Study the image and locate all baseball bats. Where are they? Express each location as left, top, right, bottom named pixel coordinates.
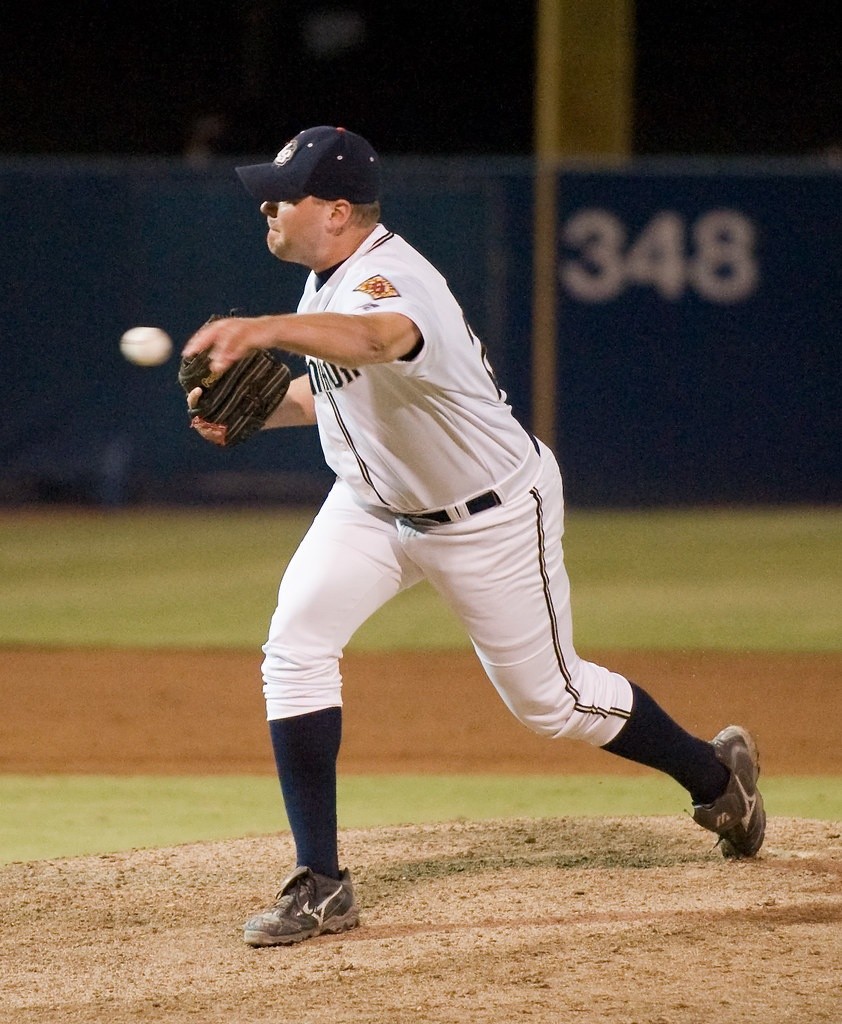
left=176, top=305, right=293, bottom=448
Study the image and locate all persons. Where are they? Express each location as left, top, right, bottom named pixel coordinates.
left=180, top=126, right=767, bottom=948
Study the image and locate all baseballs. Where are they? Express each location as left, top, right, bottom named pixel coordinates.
left=122, top=325, right=172, bottom=363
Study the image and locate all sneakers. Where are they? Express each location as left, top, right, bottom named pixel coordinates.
left=243, top=866, right=361, bottom=946
left=691, top=724, right=766, bottom=859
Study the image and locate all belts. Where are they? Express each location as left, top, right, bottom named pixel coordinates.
left=394, top=433, right=541, bottom=527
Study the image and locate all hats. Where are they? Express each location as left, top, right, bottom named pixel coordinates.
left=237, top=125, right=381, bottom=204
